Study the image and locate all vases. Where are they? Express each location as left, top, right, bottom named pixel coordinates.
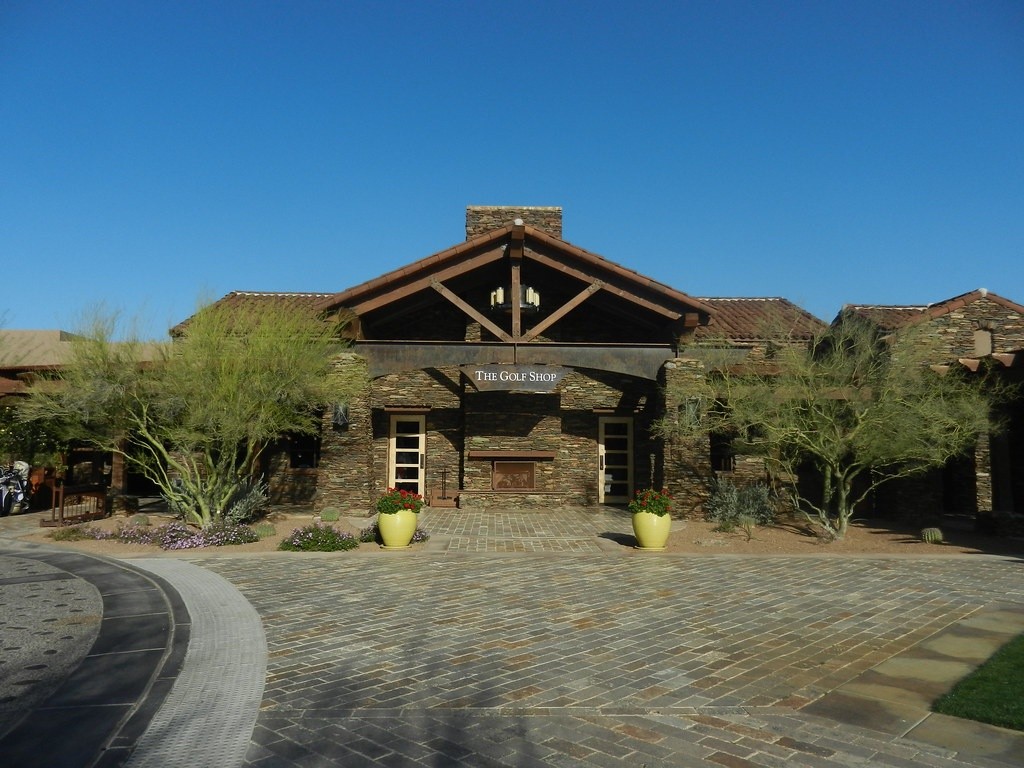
left=631, top=508, right=672, bottom=552
left=378, top=509, right=417, bottom=548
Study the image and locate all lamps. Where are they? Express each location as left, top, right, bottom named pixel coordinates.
left=490, top=286, right=541, bottom=315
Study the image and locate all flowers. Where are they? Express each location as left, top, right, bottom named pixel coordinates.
left=377, top=486, right=424, bottom=515
left=629, top=484, right=675, bottom=516
